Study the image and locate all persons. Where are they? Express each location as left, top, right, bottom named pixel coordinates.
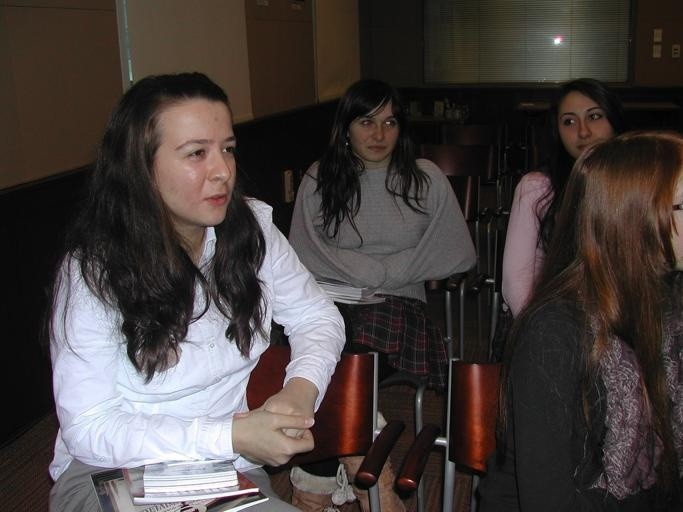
left=470, top=126, right=683, bottom=512
left=287, top=75, right=480, bottom=512
left=500, top=77, right=627, bottom=330
left=47, top=69, right=348, bottom=512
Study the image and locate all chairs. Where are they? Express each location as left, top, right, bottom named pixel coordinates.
left=229, top=98, right=683, bottom=512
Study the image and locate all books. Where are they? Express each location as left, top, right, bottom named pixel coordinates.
left=85, top=455, right=269, bottom=512
left=313, top=276, right=388, bottom=309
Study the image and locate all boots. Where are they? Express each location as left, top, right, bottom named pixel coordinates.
left=358, top=417, right=407, bottom=511
left=289, top=462, right=357, bottom=512
